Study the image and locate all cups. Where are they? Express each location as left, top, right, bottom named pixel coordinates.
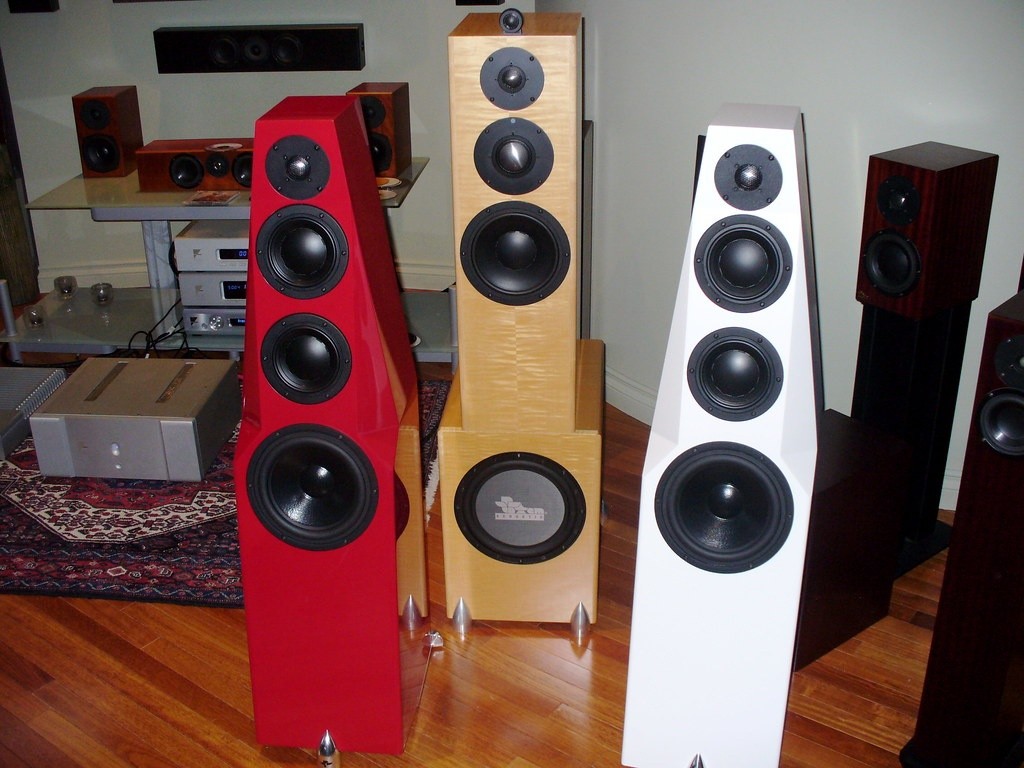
left=90, top=282, right=114, bottom=308
left=23, top=304, right=48, bottom=331
left=53, top=276, right=78, bottom=300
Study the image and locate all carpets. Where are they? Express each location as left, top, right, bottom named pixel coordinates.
left=0, top=379, right=451, bottom=610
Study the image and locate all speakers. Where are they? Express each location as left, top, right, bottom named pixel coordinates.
left=133, top=139, right=250, bottom=195
left=154, top=23, right=366, bottom=74
left=346, top=83, right=412, bottom=174
left=856, top=141, right=999, bottom=322
left=623, top=104, right=825, bottom=768
left=233, top=94, right=425, bottom=751
left=72, top=85, right=142, bottom=179
left=447, top=11, right=585, bottom=432
left=437, top=337, right=604, bottom=629
left=915, top=294, right=1024, bottom=768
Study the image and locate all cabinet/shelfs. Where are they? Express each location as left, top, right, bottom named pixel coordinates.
left=0, top=157, right=459, bottom=375
